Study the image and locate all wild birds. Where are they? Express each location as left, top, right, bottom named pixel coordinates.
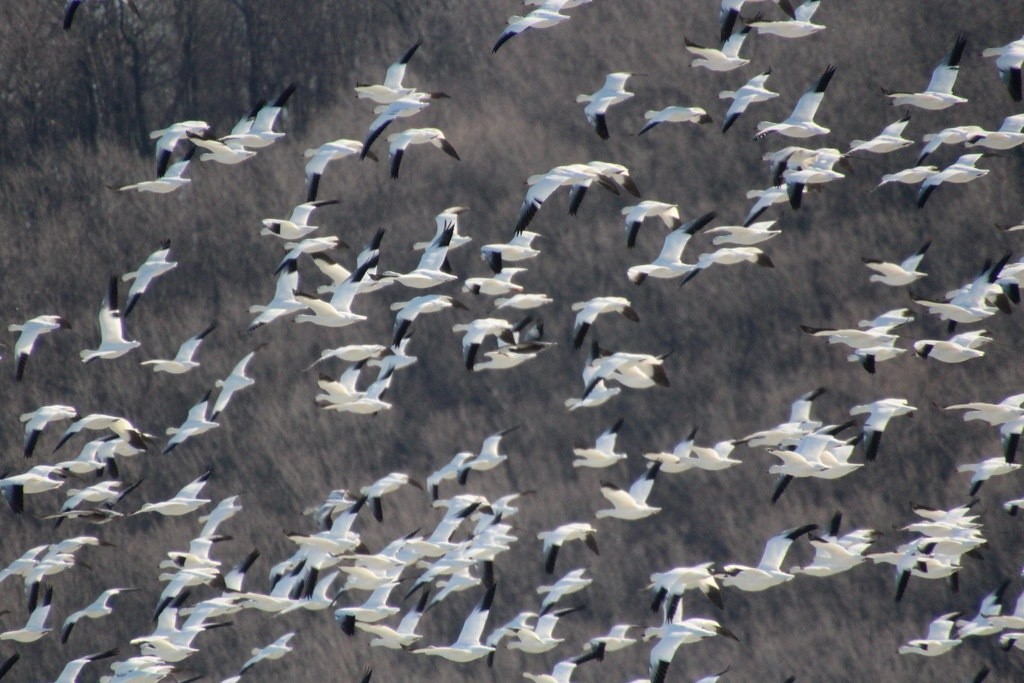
left=0, top=0, right=1023, bottom=683
